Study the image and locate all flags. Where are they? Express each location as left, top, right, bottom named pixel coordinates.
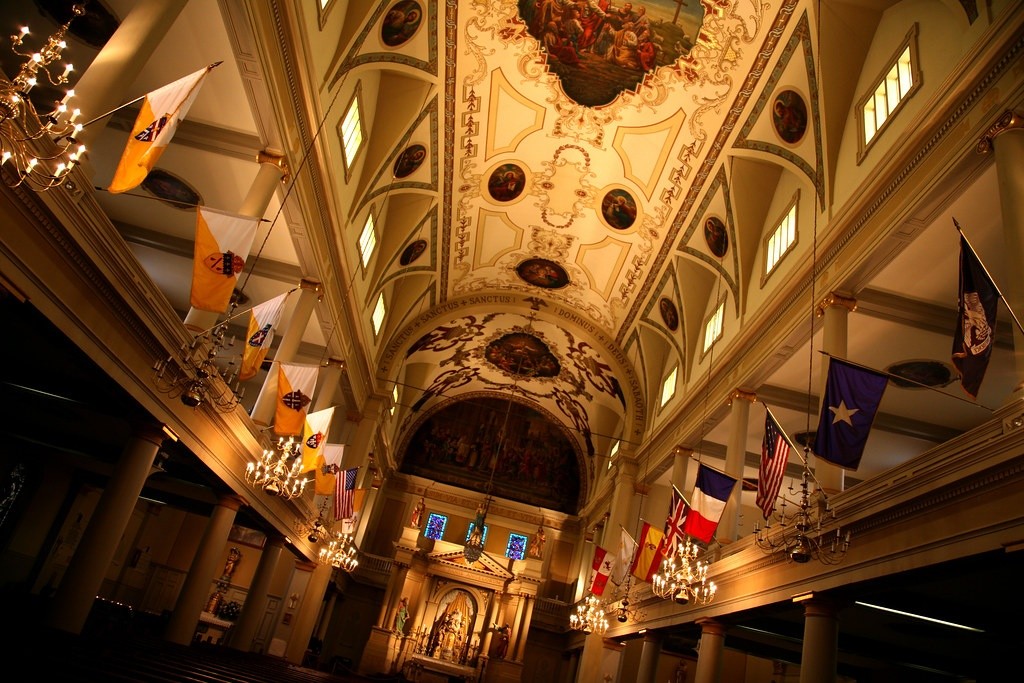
left=240, top=291, right=289, bottom=381
left=312, top=443, right=344, bottom=495
left=659, top=485, right=691, bottom=562
left=684, top=461, right=738, bottom=544
left=609, top=527, right=636, bottom=585
left=106, top=66, right=211, bottom=196
left=189, top=206, right=260, bottom=314
left=587, top=544, right=619, bottom=597
left=301, top=407, right=335, bottom=474
left=330, top=467, right=359, bottom=521
left=274, top=361, right=322, bottom=437
left=630, top=522, right=669, bottom=583
left=950, top=234, right=1000, bottom=401
left=810, top=355, right=890, bottom=472
left=753, top=409, right=792, bottom=522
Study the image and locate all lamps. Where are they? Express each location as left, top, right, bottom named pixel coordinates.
left=566, top=593, right=610, bottom=639
left=149, top=302, right=246, bottom=413
left=0, top=0, right=90, bottom=192
left=749, top=445, right=852, bottom=568
left=320, top=533, right=358, bottom=572
left=245, top=435, right=308, bottom=500
left=653, top=536, right=717, bottom=606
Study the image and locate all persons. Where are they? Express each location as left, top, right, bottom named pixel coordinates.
left=436, top=609, right=466, bottom=651
left=394, top=597, right=410, bottom=635
left=526, top=527, right=546, bottom=558
left=409, top=498, right=425, bottom=529
left=491, top=622, right=512, bottom=661
left=471, top=502, right=488, bottom=541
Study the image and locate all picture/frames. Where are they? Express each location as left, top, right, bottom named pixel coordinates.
left=229, top=525, right=266, bottom=550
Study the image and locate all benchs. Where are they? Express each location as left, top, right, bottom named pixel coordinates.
left=89, top=646, right=372, bottom=683
